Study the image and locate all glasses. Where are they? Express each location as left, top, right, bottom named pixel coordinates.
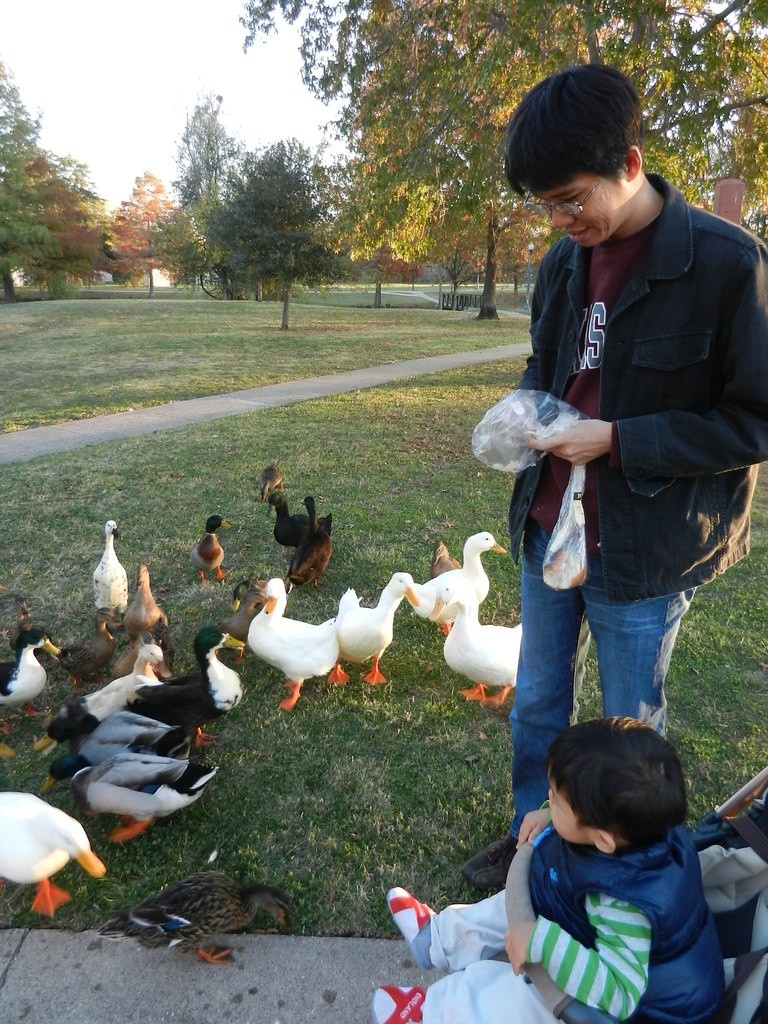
left=522, top=175, right=598, bottom=215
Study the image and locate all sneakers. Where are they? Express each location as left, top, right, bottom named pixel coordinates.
left=461, top=834, right=519, bottom=889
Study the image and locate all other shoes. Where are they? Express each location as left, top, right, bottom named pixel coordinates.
left=386, top=886, right=434, bottom=971
left=371, top=983, right=424, bottom=1024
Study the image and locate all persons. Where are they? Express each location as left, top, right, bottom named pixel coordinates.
left=459, top=63, right=768, bottom=888
left=373, top=715, right=728, bottom=1023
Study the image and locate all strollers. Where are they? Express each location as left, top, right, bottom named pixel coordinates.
left=459, top=764, right=767, bottom=1023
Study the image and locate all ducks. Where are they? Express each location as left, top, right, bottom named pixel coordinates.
left=213, top=570, right=267, bottom=665
left=247, top=578, right=339, bottom=712
left=286, top=495, right=333, bottom=595
left=53, top=608, right=124, bottom=694
left=0, top=589, right=62, bottom=736
left=429, top=575, right=523, bottom=710
left=32, top=698, right=190, bottom=768
left=1, top=790, right=108, bottom=920
left=39, top=642, right=174, bottom=722
left=190, top=514, right=233, bottom=586
left=120, top=563, right=169, bottom=647
left=325, top=571, right=424, bottom=687
left=266, top=490, right=333, bottom=550
left=38, top=753, right=220, bottom=843
left=258, top=463, right=285, bottom=504
left=94, top=869, right=293, bottom=966
left=405, top=531, right=507, bottom=637
left=120, top=626, right=248, bottom=749
left=92, top=519, right=128, bottom=623
